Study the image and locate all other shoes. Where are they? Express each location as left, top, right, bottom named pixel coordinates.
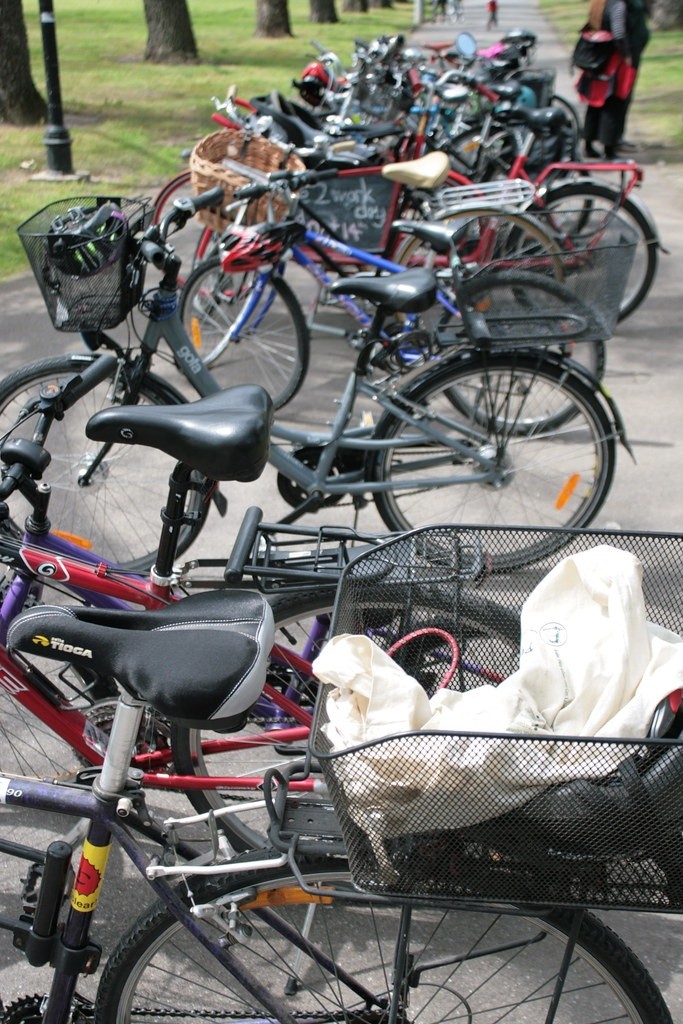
left=604, top=146, right=617, bottom=160
left=585, top=144, right=600, bottom=158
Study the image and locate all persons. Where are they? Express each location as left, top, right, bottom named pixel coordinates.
left=584, top=0, right=649, bottom=163
left=485, top=0, right=498, bottom=32
left=432, top=0, right=463, bottom=23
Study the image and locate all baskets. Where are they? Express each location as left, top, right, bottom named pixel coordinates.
left=16, top=197, right=156, bottom=332
left=306, top=524, right=683, bottom=914
left=188, top=127, right=305, bottom=236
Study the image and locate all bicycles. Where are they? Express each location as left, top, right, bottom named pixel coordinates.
left=0, top=523, right=682, bottom=1024
left=0, top=352, right=525, bottom=869
left=0, top=188, right=635, bottom=574
left=151, top=33, right=670, bottom=437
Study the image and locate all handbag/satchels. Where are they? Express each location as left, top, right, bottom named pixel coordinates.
left=573, top=24, right=618, bottom=76
left=41, top=201, right=129, bottom=326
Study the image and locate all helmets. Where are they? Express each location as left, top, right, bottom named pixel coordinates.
left=521, top=687, right=683, bottom=851
left=298, top=61, right=330, bottom=105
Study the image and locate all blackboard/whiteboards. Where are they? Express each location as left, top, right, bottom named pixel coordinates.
left=299, top=174, right=403, bottom=253
